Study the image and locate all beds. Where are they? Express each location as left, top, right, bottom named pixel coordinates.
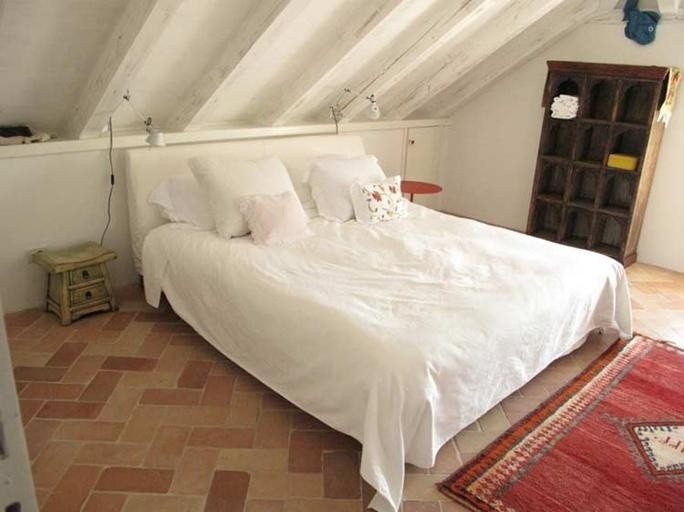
left=119, top=134, right=636, bottom=511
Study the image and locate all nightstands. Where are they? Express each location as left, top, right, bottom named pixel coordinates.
left=31, top=239, right=120, bottom=326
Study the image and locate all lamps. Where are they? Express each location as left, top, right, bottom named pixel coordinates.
left=100, top=91, right=165, bottom=150
left=328, top=87, right=381, bottom=132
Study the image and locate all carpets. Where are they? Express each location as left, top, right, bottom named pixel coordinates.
left=438, top=328, right=684, bottom=511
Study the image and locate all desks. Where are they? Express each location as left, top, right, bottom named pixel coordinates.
left=396, top=179, right=442, bottom=206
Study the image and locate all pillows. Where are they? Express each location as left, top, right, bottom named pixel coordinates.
left=302, top=153, right=387, bottom=223
left=149, top=173, right=214, bottom=231
left=234, top=187, right=315, bottom=246
left=347, top=173, right=410, bottom=225
left=183, top=151, right=310, bottom=241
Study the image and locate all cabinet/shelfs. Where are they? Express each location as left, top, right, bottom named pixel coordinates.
left=524, top=58, right=681, bottom=268
